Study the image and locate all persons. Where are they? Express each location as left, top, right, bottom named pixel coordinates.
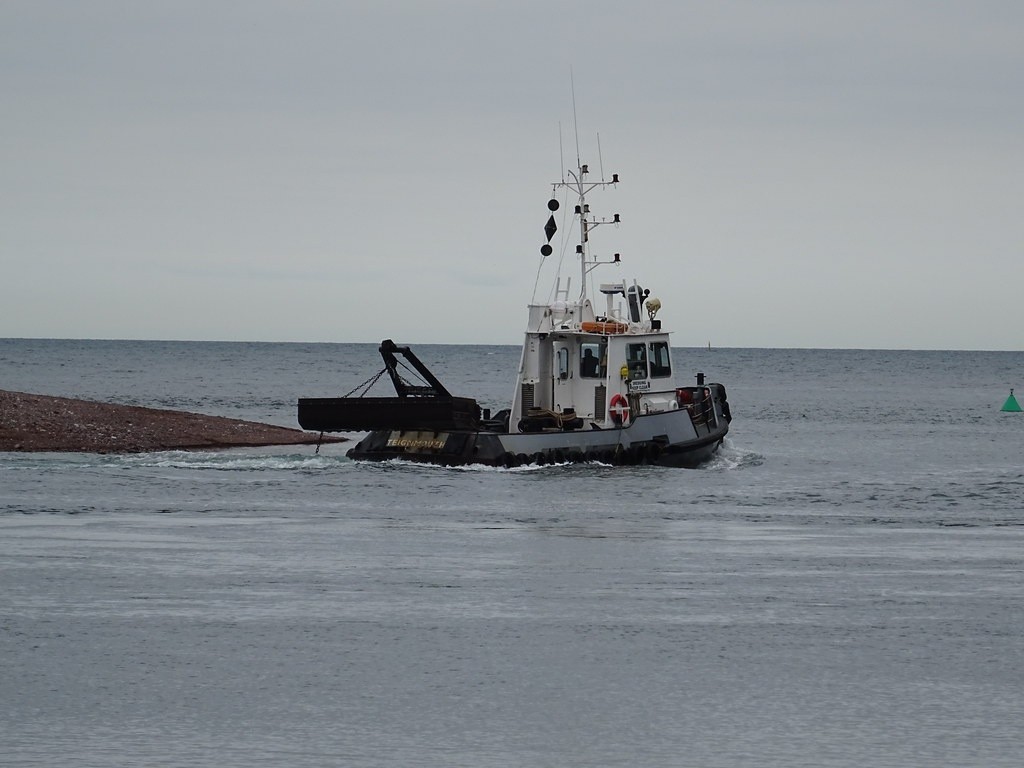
left=582, top=349, right=599, bottom=377
left=640, top=352, right=658, bottom=376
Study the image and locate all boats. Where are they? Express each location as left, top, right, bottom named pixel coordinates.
left=298, top=65, right=732, bottom=467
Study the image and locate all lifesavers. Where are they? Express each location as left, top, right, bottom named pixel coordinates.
left=582, top=320, right=629, bottom=333
left=500, top=441, right=663, bottom=468
left=609, top=394, right=628, bottom=424
